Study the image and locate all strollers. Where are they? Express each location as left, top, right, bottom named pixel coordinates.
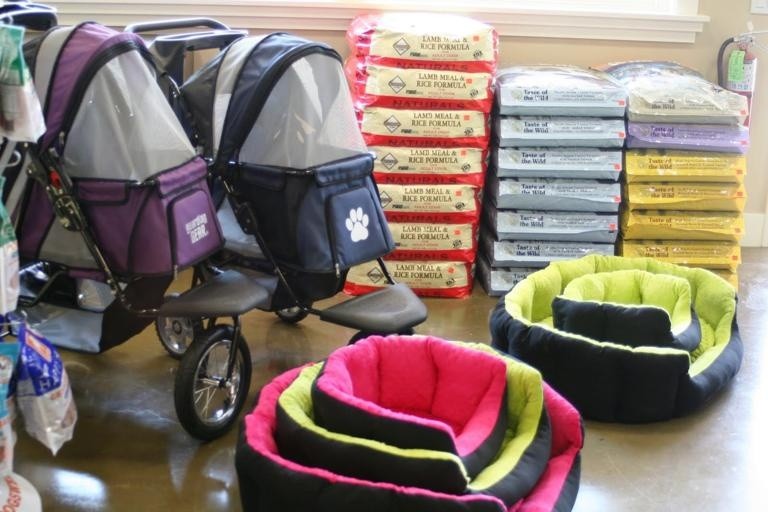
left=124, top=18, right=427, bottom=347
left=0, top=3, right=280, bottom=440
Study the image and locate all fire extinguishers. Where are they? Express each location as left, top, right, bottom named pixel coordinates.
left=717, top=22, right=768, bottom=127
